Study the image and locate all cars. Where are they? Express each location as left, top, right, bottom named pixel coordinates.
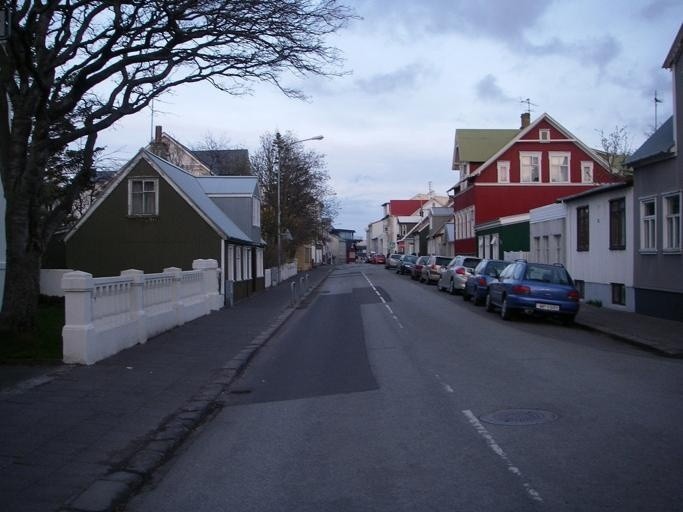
left=384, top=253, right=580, bottom=321
left=368, top=253, right=385, bottom=264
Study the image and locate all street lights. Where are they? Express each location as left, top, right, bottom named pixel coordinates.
left=277, top=135, right=324, bottom=285
